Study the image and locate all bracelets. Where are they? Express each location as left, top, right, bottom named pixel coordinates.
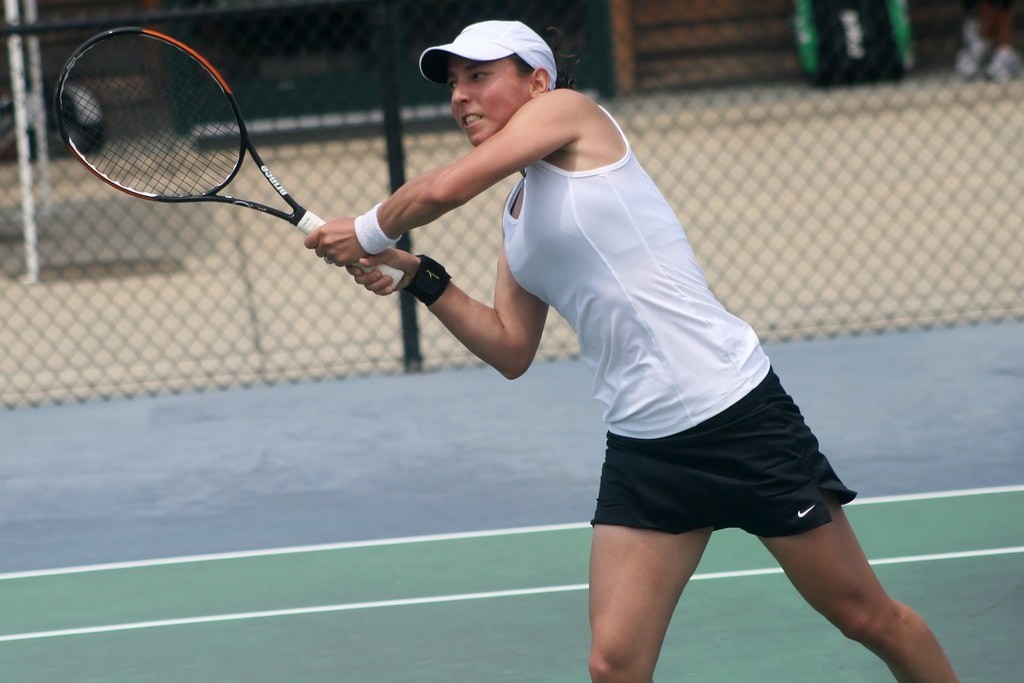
left=354, top=203, right=403, bottom=255
left=403, top=254, right=451, bottom=308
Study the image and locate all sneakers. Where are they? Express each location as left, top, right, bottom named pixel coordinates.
left=954, top=19, right=994, bottom=80
left=985, top=50, right=1021, bottom=82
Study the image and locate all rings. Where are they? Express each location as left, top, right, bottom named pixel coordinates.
left=324, top=256, right=333, bottom=264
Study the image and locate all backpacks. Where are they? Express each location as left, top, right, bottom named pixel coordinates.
left=791, top=0, right=915, bottom=87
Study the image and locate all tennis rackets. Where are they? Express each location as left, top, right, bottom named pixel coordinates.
left=53, top=25, right=406, bottom=293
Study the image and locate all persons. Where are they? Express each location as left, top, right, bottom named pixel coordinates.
left=301, top=20, right=955, bottom=683
left=956, top=0, right=1020, bottom=79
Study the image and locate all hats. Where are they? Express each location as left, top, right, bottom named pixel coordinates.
left=419, top=20, right=557, bottom=91
